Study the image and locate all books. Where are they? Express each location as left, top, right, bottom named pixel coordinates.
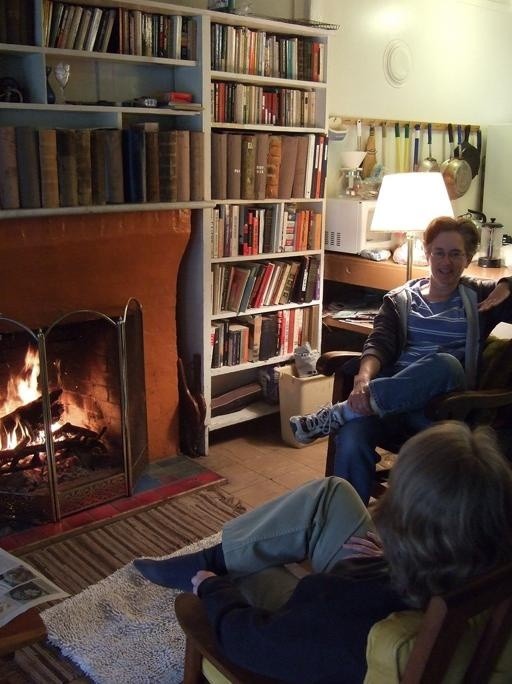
left=0, top=124, right=207, bottom=210
left=210, top=25, right=319, bottom=368
left=0, top=0, right=198, bottom=60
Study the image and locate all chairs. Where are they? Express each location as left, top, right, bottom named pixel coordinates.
left=316, top=322, right=512, bottom=499
left=174, top=565, right=512, bottom=684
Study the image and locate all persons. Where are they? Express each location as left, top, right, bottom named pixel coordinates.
left=133, top=419, right=512, bottom=684
left=290, top=218, right=512, bottom=508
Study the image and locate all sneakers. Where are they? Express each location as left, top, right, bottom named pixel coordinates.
left=289, top=402, right=346, bottom=444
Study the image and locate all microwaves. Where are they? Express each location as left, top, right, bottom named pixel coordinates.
left=325, top=197, right=403, bottom=256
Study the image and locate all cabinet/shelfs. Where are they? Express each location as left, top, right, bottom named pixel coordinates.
left=0, top=1, right=206, bottom=218
left=206, top=13, right=332, bottom=432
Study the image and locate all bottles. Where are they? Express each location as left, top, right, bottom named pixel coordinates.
left=478, top=217, right=504, bottom=268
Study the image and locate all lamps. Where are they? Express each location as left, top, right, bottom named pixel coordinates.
left=370, top=171, right=453, bottom=281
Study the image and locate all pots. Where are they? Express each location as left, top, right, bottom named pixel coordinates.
left=439, top=123, right=456, bottom=174
left=417, top=123, right=440, bottom=171
left=443, top=124, right=473, bottom=201
left=454, top=125, right=480, bottom=179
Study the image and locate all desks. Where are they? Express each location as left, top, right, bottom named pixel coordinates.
left=325, top=255, right=512, bottom=337
left=0, top=607, right=48, bottom=662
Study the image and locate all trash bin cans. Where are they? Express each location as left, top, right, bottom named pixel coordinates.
left=275, top=363, right=336, bottom=448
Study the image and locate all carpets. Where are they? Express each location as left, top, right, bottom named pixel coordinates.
left=10, top=484, right=311, bottom=684
left=40, top=529, right=223, bottom=683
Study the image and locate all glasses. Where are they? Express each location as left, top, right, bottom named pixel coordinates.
left=429, top=250, right=467, bottom=260
